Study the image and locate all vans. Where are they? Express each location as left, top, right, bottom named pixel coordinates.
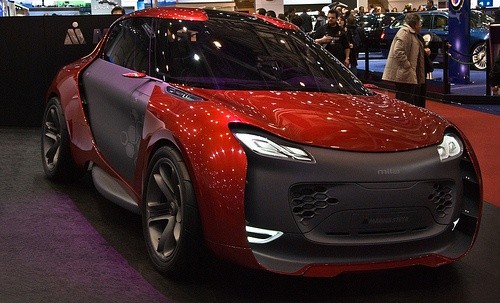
left=361, top=12, right=404, bottom=52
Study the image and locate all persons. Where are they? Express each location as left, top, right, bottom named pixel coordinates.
left=313, top=9, right=351, bottom=67
left=336, top=14, right=358, bottom=78
left=110, top=0, right=500, bottom=31
left=382, top=13, right=431, bottom=110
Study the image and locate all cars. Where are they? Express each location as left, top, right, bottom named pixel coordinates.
left=381, top=10, right=495, bottom=70
left=40, top=6, right=484, bottom=284
